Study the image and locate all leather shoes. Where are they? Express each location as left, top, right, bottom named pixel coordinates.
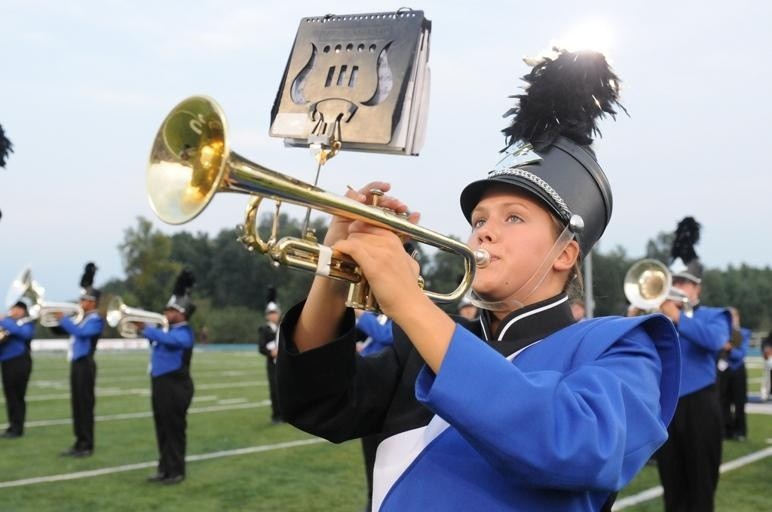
left=162, top=472, right=184, bottom=484
left=60, top=447, right=91, bottom=459
left=147, top=469, right=168, bottom=481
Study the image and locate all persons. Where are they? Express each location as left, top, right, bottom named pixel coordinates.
left=53, top=286, right=104, bottom=457
left=624, top=258, right=732, bottom=512
left=0, top=301, right=33, bottom=438
left=457, top=292, right=480, bottom=320
left=350, top=306, right=395, bottom=512
left=130, top=293, right=194, bottom=483
left=274, top=134, right=679, bottom=512
left=261, top=301, right=287, bottom=421
left=568, top=295, right=585, bottom=320
left=717, top=304, right=754, bottom=438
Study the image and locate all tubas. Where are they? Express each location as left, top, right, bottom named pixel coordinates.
left=0, top=270, right=43, bottom=338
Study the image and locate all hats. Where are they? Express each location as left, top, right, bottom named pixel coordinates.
left=164, top=266, right=196, bottom=318
left=17, top=268, right=38, bottom=310
left=459, top=44, right=618, bottom=258
left=264, top=283, right=281, bottom=315
left=669, top=216, right=704, bottom=284
left=79, top=262, right=100, bottom=301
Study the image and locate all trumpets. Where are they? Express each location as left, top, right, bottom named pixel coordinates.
left=31, top=300, right=84, bottom=330
left=624, top=259, right=694, bottom=322
left=146, top=96, right=490, bottom=317
left=107, top=296, right=170, bottom=338
